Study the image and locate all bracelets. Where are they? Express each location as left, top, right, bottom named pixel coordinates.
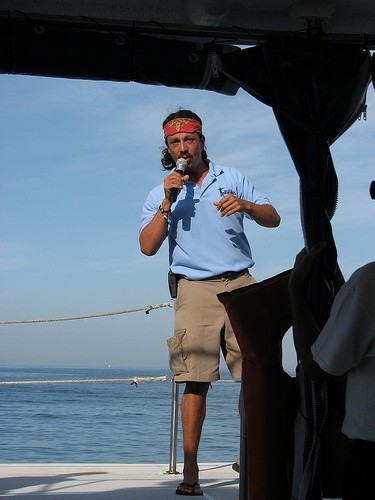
left=159, top=206, right=172, bottom=224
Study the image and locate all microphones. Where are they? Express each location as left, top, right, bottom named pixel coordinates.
left=168, top=157, right=188, bottom=203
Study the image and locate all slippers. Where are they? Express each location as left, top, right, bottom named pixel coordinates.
left=175, top=482, right=203, bottom=496
left=232, top=463, right=239, bottom=473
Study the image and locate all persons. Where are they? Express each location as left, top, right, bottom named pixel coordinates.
left=138, top=110, right=281, bottom=495
left=289, top=240, right=375, bottom=500
